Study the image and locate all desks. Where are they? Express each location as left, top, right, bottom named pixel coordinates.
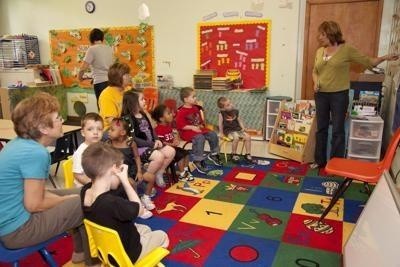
left=0, top=119, right=83, bottom=190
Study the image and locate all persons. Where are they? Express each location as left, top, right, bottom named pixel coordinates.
left=153, top=104, right=195, bottom=182
left=121, top=90, right=176, bottom=188
left=76, top=28, right=114, bottom=112
left=107, top=117, right=157, bottom=220
left=80, top=142, right=169, bottom=267
left=175, top=87, right=224, bottom=175
left=0, top=91, right=101, bottom=267
left=71, top=112, right=105, bottom=189
left=310, top=20, right=399, bottom=169
left=97, top=62, right=132, bottom=137
left=218, top=96, right=253, bottom=164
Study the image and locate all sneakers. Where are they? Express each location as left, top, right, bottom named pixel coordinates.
left=71, top=252, right=85, bottom=263
left=140, top=208, right=153, bottom=220
left=208, top=154, right=223, bottom=166
left=177, top=174, right=188, bottom=181
left=141, top=196, right=155, bottom=211
left=192, top=161, right=206, bottom=174
left=244, top=153, right=252, bottom=163
left=231, top=153, right=239, bottom=162
left=185, top=170, right=194, bottom=181
left=155, top=173, right=166, bottom=188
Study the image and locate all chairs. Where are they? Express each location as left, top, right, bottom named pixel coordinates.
left=83, top=219, right=170, bottom=267
left=0, top=231, right=69, bottom=267
left=318, top=131, right=400, bottom=222
left=62, top=157, right=74, bottom=188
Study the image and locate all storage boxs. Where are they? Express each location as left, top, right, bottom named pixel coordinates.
left=0, top=68, right=56, bottom=86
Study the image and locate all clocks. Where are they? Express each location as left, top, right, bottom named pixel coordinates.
left=85, top=1, right=95, bottom=13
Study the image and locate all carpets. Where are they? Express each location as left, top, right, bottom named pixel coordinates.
left=0, top=149, right=399, bottom=267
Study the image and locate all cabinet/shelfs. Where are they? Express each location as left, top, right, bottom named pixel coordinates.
left=346, top=111, right=384, bottom=162
left=268, top=101, right=320, bottom=165
left=266, top=96, right=292, bottom=141
left=158, top=86, right=269, bottom=137
left=0, top=84, right=67, bottom=126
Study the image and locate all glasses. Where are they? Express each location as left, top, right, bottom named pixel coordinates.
left=53, top=115, right=64, bottom=123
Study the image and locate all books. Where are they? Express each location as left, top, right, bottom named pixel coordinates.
left=211, top=77, right=233, bottom=91
